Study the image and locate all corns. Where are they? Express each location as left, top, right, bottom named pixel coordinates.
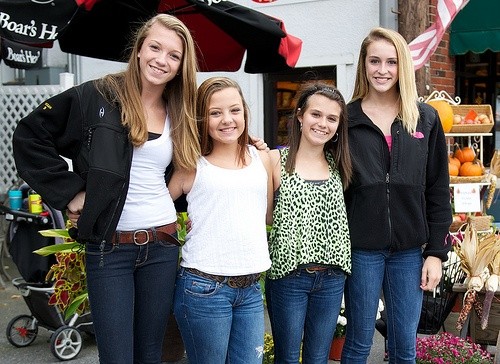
left=486, top=180, right=496, bottom=209
left=454, top=289, right=500, bottom=331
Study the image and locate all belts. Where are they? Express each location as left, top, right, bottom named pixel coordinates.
left=301, top=264, right=329, bottom=274
left=184, top=268, right=261, bottom=290
left=112, top=221, right=183, bottom=252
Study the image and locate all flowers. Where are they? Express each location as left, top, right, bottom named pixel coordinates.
left=333, top=307, right=347, bottom=337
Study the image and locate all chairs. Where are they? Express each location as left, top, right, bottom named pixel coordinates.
left=376, top=258, right=466, bottom=361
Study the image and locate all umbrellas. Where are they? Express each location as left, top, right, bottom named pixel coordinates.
left=0, top=0, right=303, bottom=76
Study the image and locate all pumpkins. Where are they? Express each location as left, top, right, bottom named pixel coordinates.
left=428, top=101, right=454, bottom=133
left=448, top=143, right=483, bottom=176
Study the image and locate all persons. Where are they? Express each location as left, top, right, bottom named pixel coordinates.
left=182, top=83, right=352, bottom=364
left=326, top=27, right=453, bottom=364
left=65, top=77, right=274, bottom=364
left=12, top=13, right=271, bottom=364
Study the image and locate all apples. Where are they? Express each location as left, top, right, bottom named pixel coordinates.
left=454, top=114, right=491, bottom=124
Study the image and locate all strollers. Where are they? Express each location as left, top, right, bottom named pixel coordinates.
left=0, top=184, right=96, bottom=361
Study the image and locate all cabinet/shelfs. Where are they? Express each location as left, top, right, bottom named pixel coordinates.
left=276, top=87, right=294, bottom=144
left=417, top=89, right=495, bottom=241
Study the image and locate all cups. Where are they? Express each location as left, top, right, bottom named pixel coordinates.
left=8, top=190, right=22, bottom=210
left=28, top=194, right=42, bottom=214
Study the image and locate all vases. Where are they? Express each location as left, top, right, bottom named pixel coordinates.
left=329, top=336, right=346, bottom=360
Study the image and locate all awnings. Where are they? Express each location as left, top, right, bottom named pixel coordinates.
left=449, top=0, right=500, bottom=55
